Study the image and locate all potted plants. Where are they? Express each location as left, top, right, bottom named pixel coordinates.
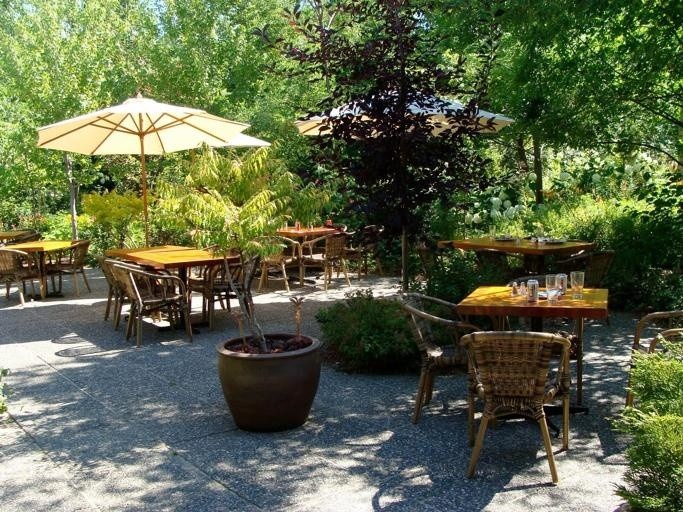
left=148, top=138, right=325, bottom=434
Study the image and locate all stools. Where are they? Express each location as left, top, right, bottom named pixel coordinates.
left=624, top=304, right=683, bottom=420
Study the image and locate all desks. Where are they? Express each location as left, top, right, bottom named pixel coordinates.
left=452, top=285, right=610, bottom=442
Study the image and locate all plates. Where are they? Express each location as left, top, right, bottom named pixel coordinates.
left=494, top=238, right=517, bottom=241
left=542, top=239, right=567, bottom=244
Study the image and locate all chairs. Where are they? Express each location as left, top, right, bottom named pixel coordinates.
left=416, top=236, right=614, bottom=289
left=454, top=329, right=577, bottom=489
left=501, top=272, right=594, bottom=408
left=94, top=244, right=261, bottom=348
left=0, top=230, right=91, bottom=306
left=247, top=223, right=384, bottom=293
left=394, top=291, right=486, bottom=428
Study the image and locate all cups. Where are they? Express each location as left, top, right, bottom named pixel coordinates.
left=280, top=221, right=287, bottom=231
left=570, top=271, right=584, bottom=299
left=489, top=225, right=496, bottom=239
left=325, top=220, right=331, bottom=228
left=306, top=221, right=312, bottom=231
left=545, top=273, right=559, bottom=303
left=294, top=219, right=300, bottom=231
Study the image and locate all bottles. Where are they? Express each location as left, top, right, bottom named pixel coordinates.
left=511, top=282, right=518, bottom=296
left=519, top=282, right=526, bottom=297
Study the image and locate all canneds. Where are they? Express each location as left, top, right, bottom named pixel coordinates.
left=527, top=280, right=539, bottom=302
left=295, top=222, right=301, bottom=231
left=555, top=273, right=567, bottom=293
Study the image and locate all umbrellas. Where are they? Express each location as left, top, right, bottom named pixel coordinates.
left=34, top=92, right=252, bottom=248
left=293, top=90, right=516, bottom=138
left=223, top=133, right=272, bottom=147
